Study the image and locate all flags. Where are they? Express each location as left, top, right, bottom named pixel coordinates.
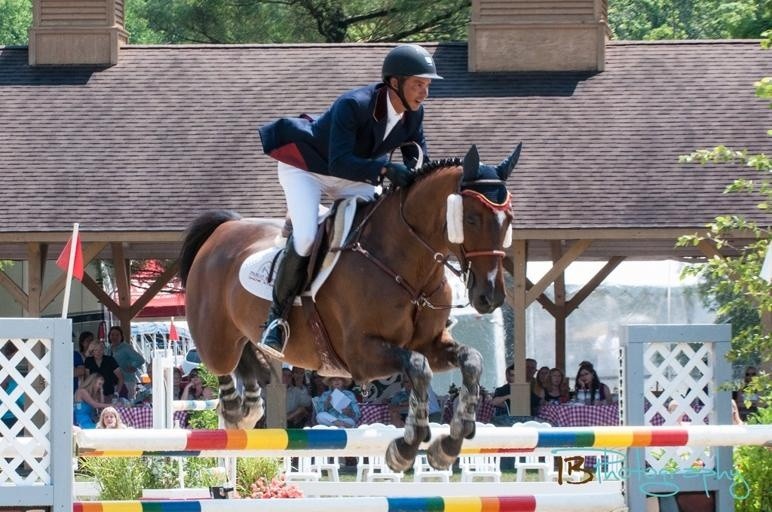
left=55, top=232, right=85, bottom=282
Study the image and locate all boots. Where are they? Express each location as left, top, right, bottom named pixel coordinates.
left=260, top=236, right=310, bottom=351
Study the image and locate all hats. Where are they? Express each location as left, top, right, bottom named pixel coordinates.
left=322, top=375, right=353, bottom=388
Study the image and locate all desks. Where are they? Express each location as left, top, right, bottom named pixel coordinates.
left=114, top=379, right=708, bottom=428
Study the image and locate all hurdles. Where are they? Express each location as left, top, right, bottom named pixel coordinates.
left=0, top=426, right=772, bottom=511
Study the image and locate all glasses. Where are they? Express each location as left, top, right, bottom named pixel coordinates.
left=190, top=373, right=202, bottom=378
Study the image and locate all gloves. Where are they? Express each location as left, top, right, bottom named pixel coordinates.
left=384, top=163, right=415, bottom=186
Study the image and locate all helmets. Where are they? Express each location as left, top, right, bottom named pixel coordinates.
left=382, top=44, right=444, bottom=80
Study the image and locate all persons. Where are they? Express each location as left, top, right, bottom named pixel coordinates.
left=72, top=326, right=765, bottom=432
left=255, top=44, right=445, bottom=357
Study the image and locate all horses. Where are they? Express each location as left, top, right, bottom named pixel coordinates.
left=177, top=140, right=524, bottom=474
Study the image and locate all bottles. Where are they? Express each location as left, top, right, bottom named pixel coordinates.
left=577, top=389, right=600, bottom=406
left=111, top=386, right=119, bottom=405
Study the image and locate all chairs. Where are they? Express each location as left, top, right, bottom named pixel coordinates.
left=284, top=422, right=585, bottom=485
